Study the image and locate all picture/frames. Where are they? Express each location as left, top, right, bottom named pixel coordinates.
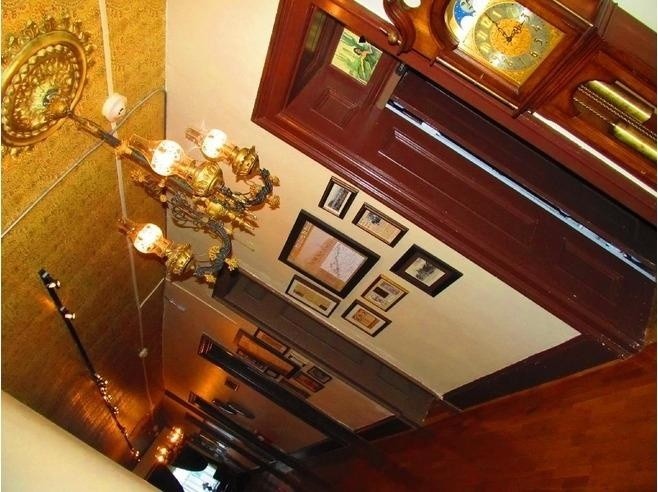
left=232, top=176, right=464, bottom=401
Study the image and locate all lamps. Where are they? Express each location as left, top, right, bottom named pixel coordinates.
left=146, top=425, right=187, bottom=467
left=1, top=15, right=279, bottom=287
left=38, top=267, right=141, bottom=462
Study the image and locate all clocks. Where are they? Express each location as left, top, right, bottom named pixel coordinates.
left=383, top=0, right=657, bottom=225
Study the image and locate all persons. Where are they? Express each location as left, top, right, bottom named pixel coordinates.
left=354, top=46, right=375, bottom=82
